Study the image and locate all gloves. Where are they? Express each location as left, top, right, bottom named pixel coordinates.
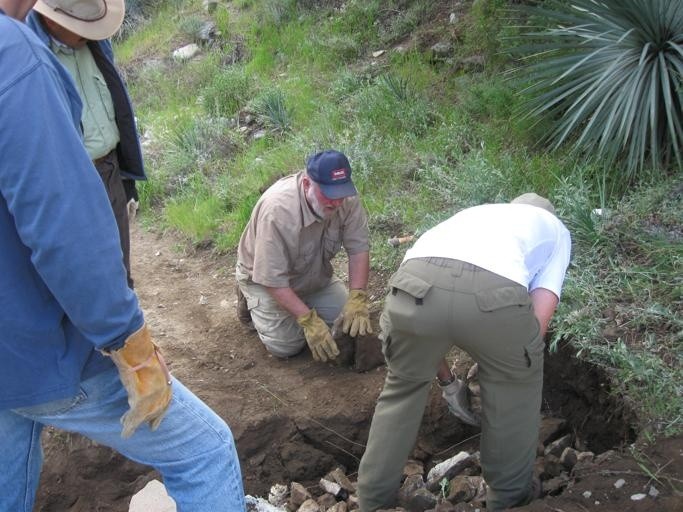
left=294, top=308, right=340, bottom=362
left=95, top=321, right=173, bottom=439
left=438, top=363, right=480, bottom=428
left=333, top=289, right=373, bottom=335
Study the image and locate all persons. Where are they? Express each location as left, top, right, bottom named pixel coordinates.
left=355, top=191, right=573, bottom=511
left=21, top=2, right=149, bottom=293
left=0, top=1, right=248, bottom=512
left=232, top=149, right=378, bottom=365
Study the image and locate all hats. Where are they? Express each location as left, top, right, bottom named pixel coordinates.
left=509, top=191, right=557, bottom=213
left=31, top=1, right=125, bottom=40
left=306, top=149, right=357, bottom=201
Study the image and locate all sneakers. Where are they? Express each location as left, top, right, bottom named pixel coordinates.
left=532, top=474, right=541, bottom=500
left=333, top=329, right=386, bottom=370
left=233, top=283, right=252, bottom=323
